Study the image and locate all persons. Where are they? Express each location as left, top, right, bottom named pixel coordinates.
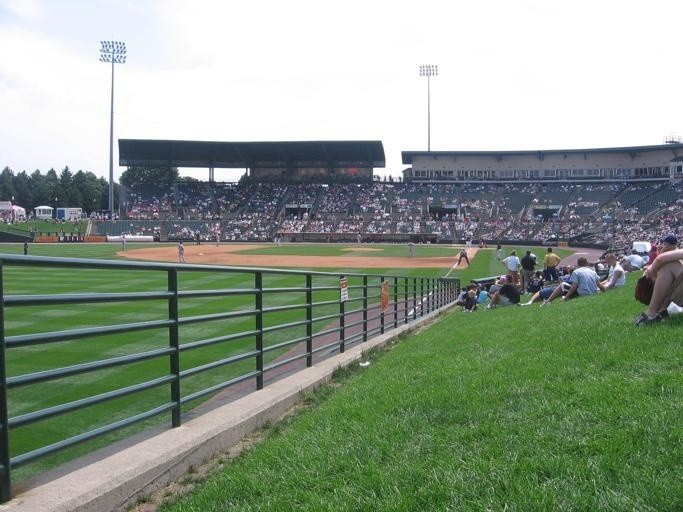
left=178, top=241, right=186, bottom=263
left=634, top=236, right=683, bottom=326
left=0, top=181, right=683, bottom=252
left=24, top=241, right=29, bottom=255
left=407, top=240, right=415, bottom=257
left=457, top=244, right=644, bottom=312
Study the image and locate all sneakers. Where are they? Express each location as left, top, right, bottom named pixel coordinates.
left=635, top=301, right=683, bottom=325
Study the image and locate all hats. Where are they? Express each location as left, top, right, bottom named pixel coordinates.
left=662, top=236, right=676, bottom=244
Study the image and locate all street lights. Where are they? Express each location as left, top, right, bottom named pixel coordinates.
left=418, top=64, right=439, bottom=152
left=93, top=197, right=96, bottom=212
left=11, top=195, right=15, bottom=207
left=95, top=37, right=128, bottom=218
left=54, top=196, right=57, bottom=209
